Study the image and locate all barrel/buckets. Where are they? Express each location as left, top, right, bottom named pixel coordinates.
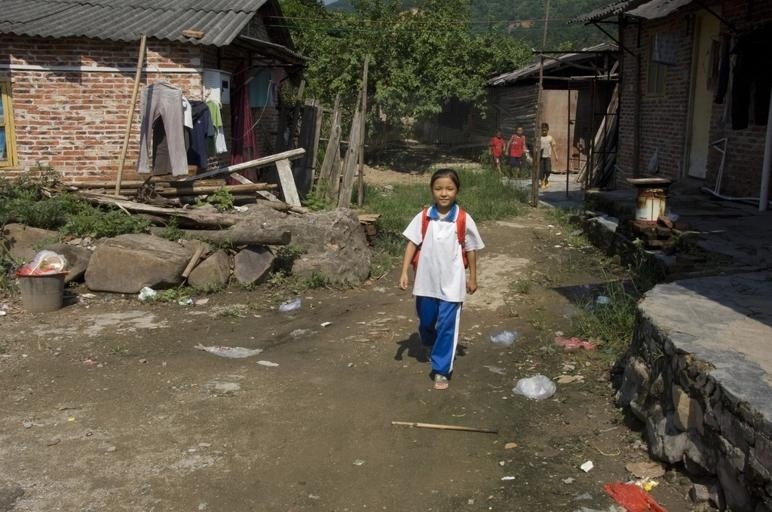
left=16, top=262, right=71, bottom=313
left=635, top=186, right=671, bottom=222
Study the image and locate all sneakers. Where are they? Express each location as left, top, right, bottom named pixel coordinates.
left=431, top=373, right=449, bottom=390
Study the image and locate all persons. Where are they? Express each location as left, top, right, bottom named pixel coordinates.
left=538, top=123, right=558, bottom=189
left=399, top=169, right=485, bottom=390
left=488, top=131, right=506, bottom=177
left=505, top=127, right=528, bottom=179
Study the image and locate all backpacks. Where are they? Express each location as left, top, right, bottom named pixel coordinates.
left=412, top=207, right=468, bottom=271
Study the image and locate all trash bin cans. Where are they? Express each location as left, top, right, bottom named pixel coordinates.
left=17, top=272, right=68, bottom=313
